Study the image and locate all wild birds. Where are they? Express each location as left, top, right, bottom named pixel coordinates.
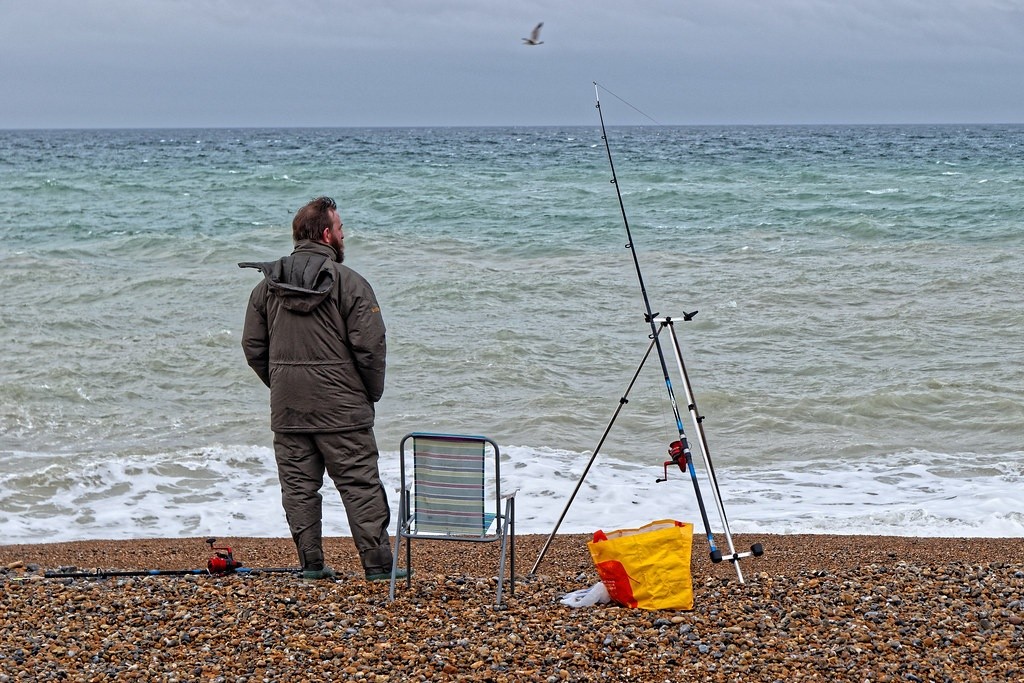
left=521, top=22, right=545, bottom=45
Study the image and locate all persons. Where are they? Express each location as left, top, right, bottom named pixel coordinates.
left=241, top=196, right=415, bottom=582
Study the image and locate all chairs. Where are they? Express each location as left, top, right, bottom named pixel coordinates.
left=389, top=432, right=520, bottom=605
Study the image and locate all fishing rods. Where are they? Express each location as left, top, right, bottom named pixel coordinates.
left=42, top=539, right=308, bottom=579
left=593, top=83, right=721, bottom=562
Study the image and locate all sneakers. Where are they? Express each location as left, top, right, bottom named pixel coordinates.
left=365, top=566, right=415, bottom=581
left=303, top=565, right=336, bottom=580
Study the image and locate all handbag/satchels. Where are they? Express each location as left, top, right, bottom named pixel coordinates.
left=587, top=519, right=694, bottom=611
